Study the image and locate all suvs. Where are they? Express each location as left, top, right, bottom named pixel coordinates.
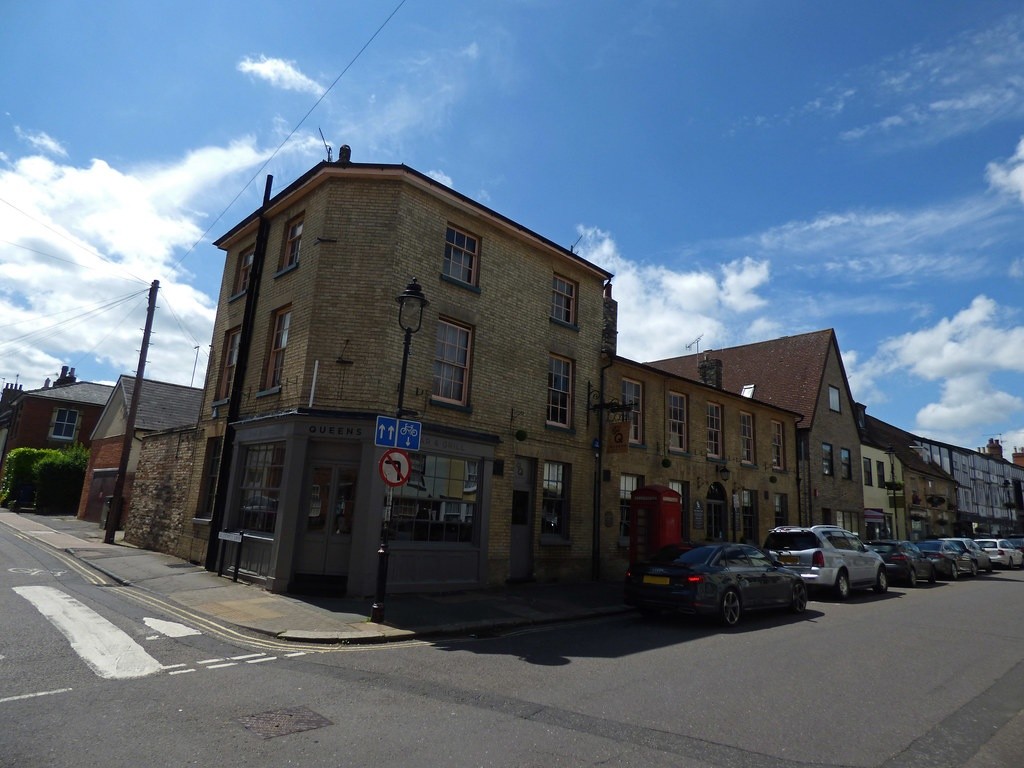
left=761, top=522, right=889, bottom=602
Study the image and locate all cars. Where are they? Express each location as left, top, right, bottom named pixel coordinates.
left=974, top=538, right=1024, bottom=570
left=938, top=537, right=992, bottom=573
left=913, top=540, right=979, bottom=581
left=861, top=539, right=940, bottom=588
left=622, top=539, right=809, bottom=627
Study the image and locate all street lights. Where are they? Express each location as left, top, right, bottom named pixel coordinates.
left=999, top=480, right=1013, bottom=535
left=369, top=277, right=431, bottom=623
left=884, top=444, right=903, bottom=539
